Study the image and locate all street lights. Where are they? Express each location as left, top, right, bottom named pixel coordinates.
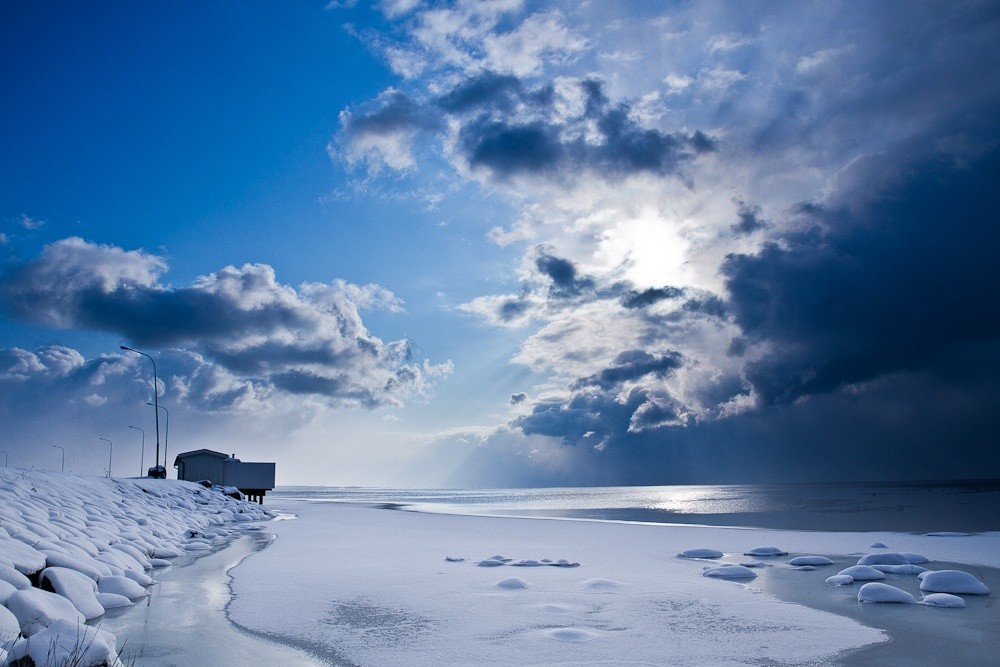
left=99, top=438, right=112, bottom=479
left=146, top=403, right=168, bottom=467
left=120, top=346, right=159, bottom=467
left=129, top=426, right=144, bottom=477
left=53, top=446, right=64, bottom=472
